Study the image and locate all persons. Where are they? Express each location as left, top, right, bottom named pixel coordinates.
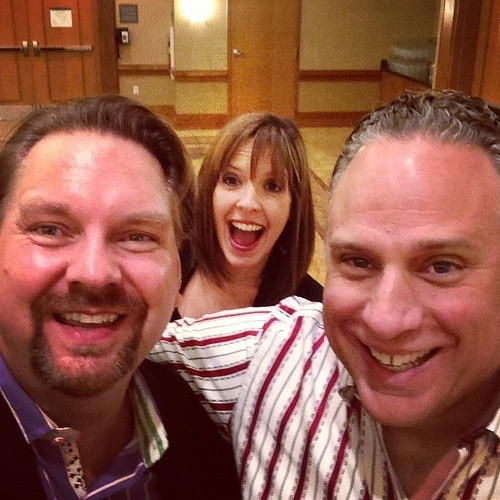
left=167, top=109, right=327, bottom=325
left=142, top=87, right=499, bottom=500
left=0, top=94, right=243, bottom=500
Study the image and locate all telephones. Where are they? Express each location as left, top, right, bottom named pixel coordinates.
left=115, top=26, right=131, bottom=45
